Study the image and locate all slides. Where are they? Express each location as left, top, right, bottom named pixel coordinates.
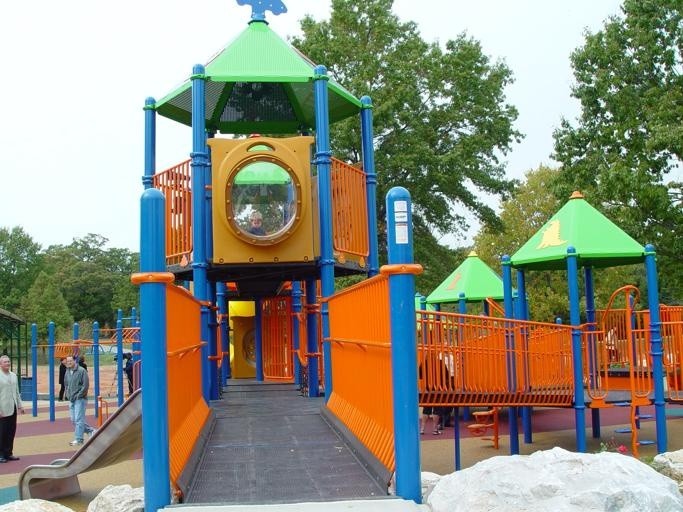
left=18, top=385, right=143, bottom=500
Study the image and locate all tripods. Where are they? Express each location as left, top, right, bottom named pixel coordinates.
left=107, top=366, right=133, bottom=398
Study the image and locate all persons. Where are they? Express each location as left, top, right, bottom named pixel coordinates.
left=287, top=201, right=296, bottom=224
left=418, top=347, right=455, bottom=436
left=123, top=353, right=134, bottom=395
left=247, top=212, right=268, bottom=236
left=58, top=358, right=68, bottom=401
left=0, top=355, right=25, bottom=463
left=64, top=356, right=99, bottom=446
left=79, top=357, right=86, bottom=371
left=605, top=326, right=619, bottom=364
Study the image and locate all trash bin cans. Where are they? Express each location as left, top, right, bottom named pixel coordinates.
left=21, top=377, right=32, bottom=401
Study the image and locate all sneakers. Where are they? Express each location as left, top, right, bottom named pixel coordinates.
left=88, top=428, right=97, bottom=439
left=69, top=439, right=83, bottom=446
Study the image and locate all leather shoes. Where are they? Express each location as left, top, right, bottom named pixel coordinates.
left=0, top=454, right=20, bottom=463
